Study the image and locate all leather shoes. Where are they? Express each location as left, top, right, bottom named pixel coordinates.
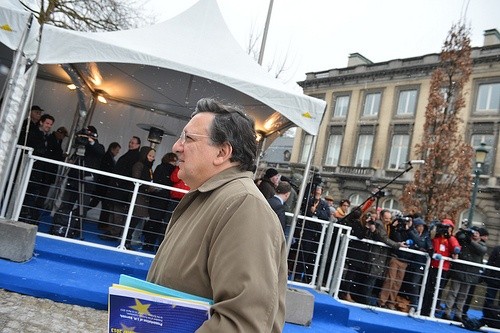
left=98, top=226, right=113, bottom=231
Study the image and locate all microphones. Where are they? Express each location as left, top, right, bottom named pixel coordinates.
left=405, top=160, right=425, bottom=165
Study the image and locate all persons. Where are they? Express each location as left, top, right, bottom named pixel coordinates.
left=0, top=97, right=191, bottom=254
left=146, top=98, right=288, bottom=333
left=253, top=168, right=500, bottom=329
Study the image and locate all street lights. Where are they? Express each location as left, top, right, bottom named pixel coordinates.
left=465, top=140, right=490, bottom=230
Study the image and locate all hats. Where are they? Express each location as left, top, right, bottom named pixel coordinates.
left=280, top=175, right=291, bottom=183
left=325, top=195, right=334, bottom=201
left=471, top=226, right=481, bottom=235
left=57, top=127, right=69, bottom=137
left=265, top=168, right=279, bottom=178
left=29, top=106, right=44, bottom=111
left=479, top=228, right=489, bottom=235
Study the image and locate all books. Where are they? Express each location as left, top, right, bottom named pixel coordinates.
left=108, top=274, right=214, bottom=333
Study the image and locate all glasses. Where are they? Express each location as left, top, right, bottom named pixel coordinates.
left=181, top=130, right=207, bottom=145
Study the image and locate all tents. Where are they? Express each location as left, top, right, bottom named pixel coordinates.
left=0, top=0, right=41, bottom=120
left=0, top=0, right=326, bottom=257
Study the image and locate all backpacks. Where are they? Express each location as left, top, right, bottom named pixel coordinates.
left=50, top=207, right=80, bottom=238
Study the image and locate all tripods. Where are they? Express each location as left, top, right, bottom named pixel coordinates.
left=34, top=144, right=84, bottom=240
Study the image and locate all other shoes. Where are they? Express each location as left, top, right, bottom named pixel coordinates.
left=340, top=294, right=354, bottom=302
left=386, top=307, right=395, bottom=310
left=441, top=312, right=451, bottom=320
left=408, top=308, right=415, bottom=315
left=452, top=315, right=463, bottom=323
left=378, top=303, right=385, bottom=308
